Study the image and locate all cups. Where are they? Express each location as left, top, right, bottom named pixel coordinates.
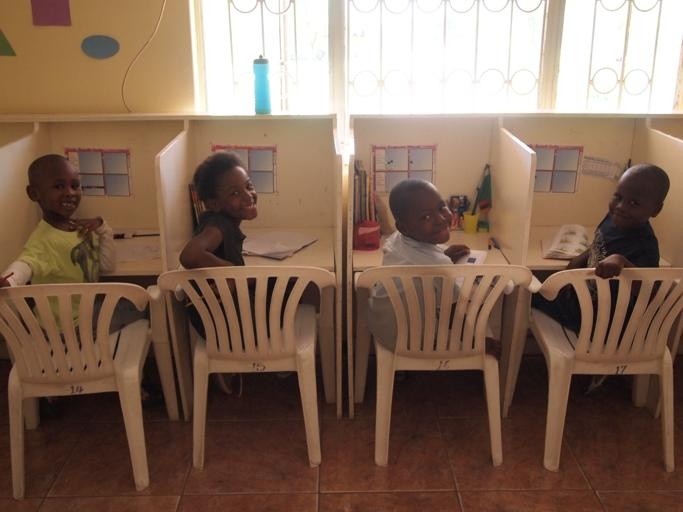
left=462, top=210, right=479, bottom=233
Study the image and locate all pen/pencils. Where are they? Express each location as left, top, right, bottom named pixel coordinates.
left=488, top=236, right=500, bottom=251
left=2, top=272, right=14, bottom=281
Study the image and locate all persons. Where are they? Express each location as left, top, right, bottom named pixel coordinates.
left=1, top=153, right=162, bottom=418
left=177, top=152, right=303, bottom=399
left=367, top=177, right=503, bottom=362
left=531, top=164, right=671, bottom=396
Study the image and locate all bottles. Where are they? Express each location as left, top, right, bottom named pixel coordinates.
left=253, top=55, right=271, bottom=113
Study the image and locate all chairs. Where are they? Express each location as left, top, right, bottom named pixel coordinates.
left=530, top=263, right=683, bottom=474
left=156, top=264, right=337, bottom=469
left=1, top=281, right=178, bottom=499
left=353, top=263, right=533, bottom=467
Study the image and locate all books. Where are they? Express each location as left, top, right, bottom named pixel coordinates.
left=538, top=223, right=596, bottom=261
left=437, top=242, right=488, bottom=288
left=187, top=184, right=206, bottom=226
left=241, top=229, right=320, bottom=260
left=353, top=159, right=379, bottom=226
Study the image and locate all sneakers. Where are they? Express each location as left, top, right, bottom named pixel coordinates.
left=585, top=375, right=613, bottom=396
left=216, top=373, right=234, bottom=396
left=141, top=386, right=164, bottom=405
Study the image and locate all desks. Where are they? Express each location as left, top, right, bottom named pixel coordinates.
left=164, top=233, right=345, bottom=418
left=527, top=224, right=680, bottom=277
left=100, top=232, right=166, bottom=279
left=345, top=225, right=521, bottom=421
